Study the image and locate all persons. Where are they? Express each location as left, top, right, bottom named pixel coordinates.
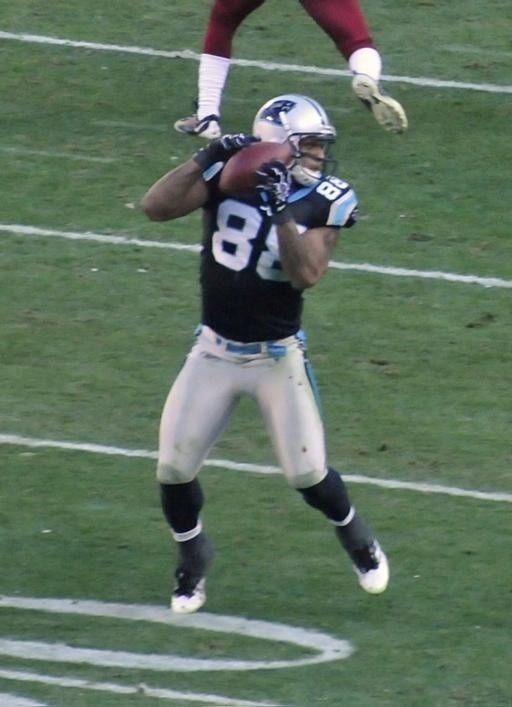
left=136, top=92, right=391, bottom=616
left=173, top=0, right=408, bottom=140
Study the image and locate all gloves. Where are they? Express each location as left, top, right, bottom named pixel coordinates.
left=254, top=160, right=292, bottom=225
left=191, top=133, right=261, bottom=168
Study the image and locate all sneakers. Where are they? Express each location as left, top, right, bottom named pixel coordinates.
left=171, top=543, right=215, bottom=614
left=352, top=74, right=408, bottom=135
left=174, top=115, right=222, bottom=139
left=337, top=523, right=390, bottom=593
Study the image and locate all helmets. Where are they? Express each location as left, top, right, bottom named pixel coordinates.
left=253, top=95, right=337, bottom=188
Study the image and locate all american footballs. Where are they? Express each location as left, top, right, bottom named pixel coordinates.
left=218, top=142, right=292, bottom=194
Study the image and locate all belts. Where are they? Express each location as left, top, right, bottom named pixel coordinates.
left=193, top=324, right=305, bottom=356
